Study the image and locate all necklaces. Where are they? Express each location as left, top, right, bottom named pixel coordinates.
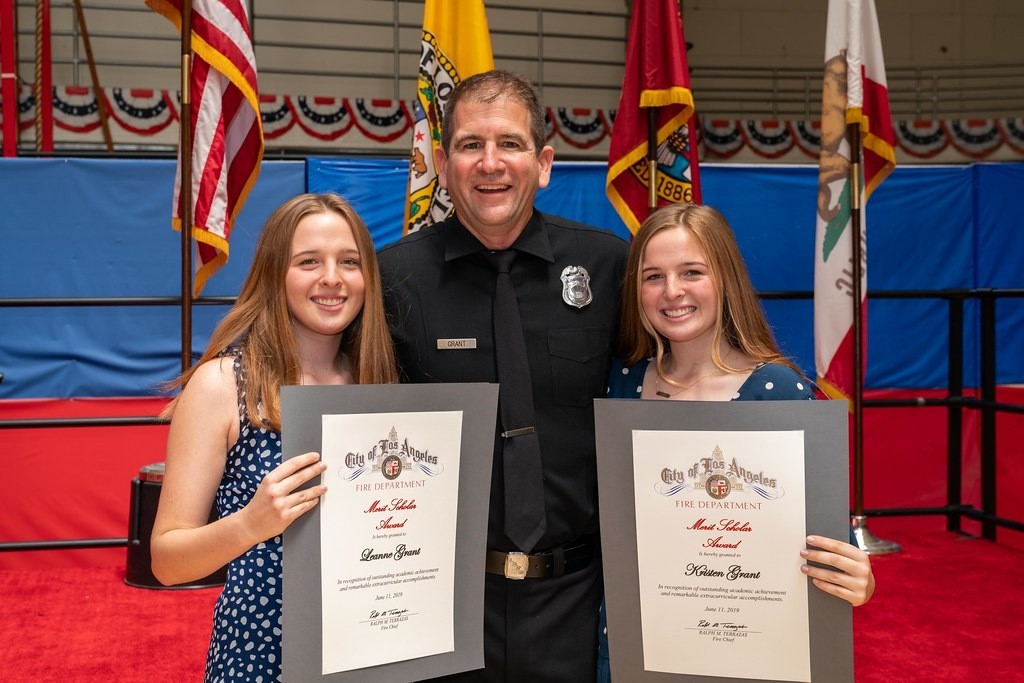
left=656, top=347, right=732, bottom=398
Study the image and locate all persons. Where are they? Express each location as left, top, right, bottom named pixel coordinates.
left=150, top=193, right=403, bottom=682
left=595, top=203, right=876, bottom=683
left=341, top=71, right=632, bottom=682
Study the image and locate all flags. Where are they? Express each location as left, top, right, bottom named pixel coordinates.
left=406, top=0, right=494, bottom=236
left=605, top=0, right=696, bottom=239
left=814, top=0, right=896, bottom=414
left=145, top=0, right=264, bottom=298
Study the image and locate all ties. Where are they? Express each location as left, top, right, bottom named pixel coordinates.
left=483, top=251, right=547, bottom=554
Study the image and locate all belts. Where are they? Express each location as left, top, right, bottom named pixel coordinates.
left=485, top=539, right=600, bottom=580
